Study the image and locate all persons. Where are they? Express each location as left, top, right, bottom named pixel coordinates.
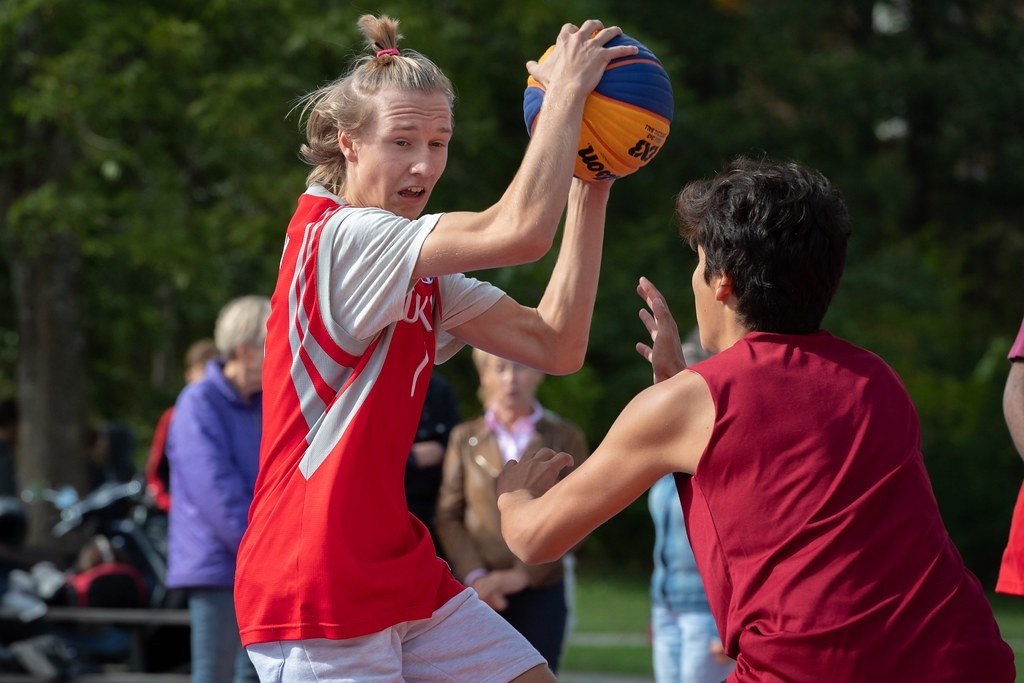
left=495, top=158, right=1024, bottom=683
left=145, top=297, right=588, bottom=683
left=235, top=14, right=640, bottom=683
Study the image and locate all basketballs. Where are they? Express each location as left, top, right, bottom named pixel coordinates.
left=522, top=30, right=676, bottom=186
